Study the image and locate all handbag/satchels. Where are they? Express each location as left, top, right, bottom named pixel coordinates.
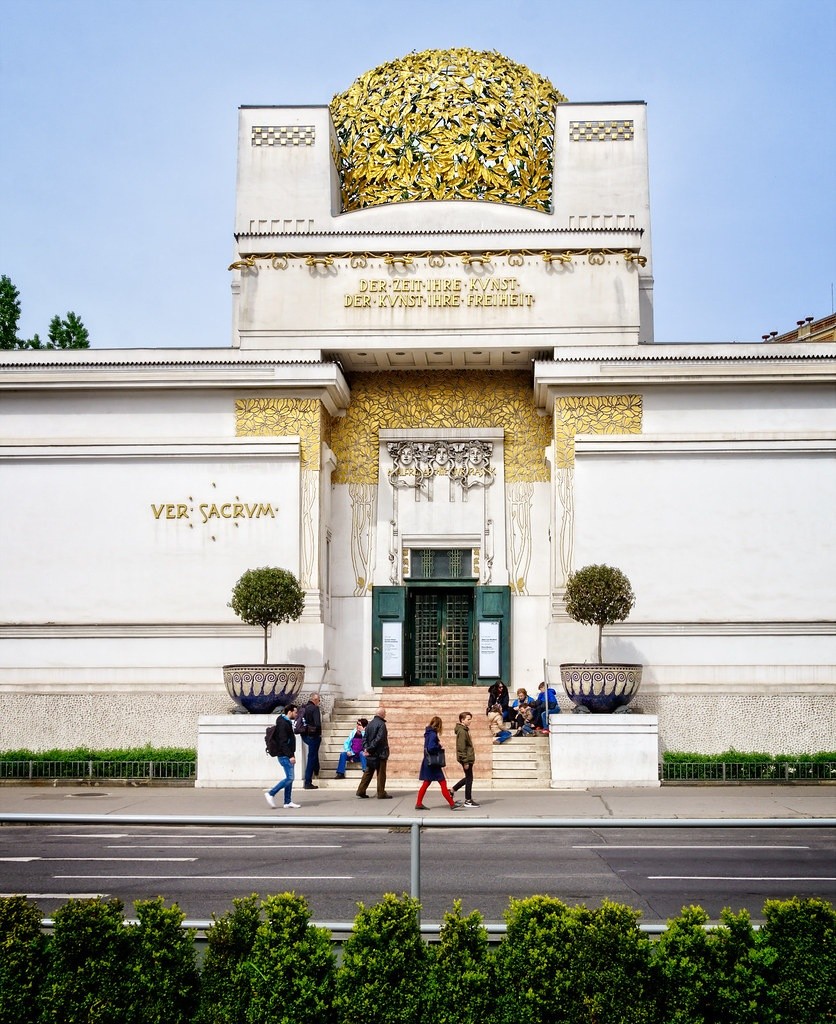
left=366, top=756, right=378, bottom=768
left=424, top=733, right=446, bottom=768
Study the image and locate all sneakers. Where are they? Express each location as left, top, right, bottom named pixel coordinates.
left=448, top=789, right=454, bottom=797
left=464, top=800, right=480, bottom=807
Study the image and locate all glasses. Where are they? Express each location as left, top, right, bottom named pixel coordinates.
left=357, top=722, right=362, bottom=726
left=498, top=686, right=503, bottom=689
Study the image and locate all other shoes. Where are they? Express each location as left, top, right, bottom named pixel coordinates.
left=513, top=730, right=523, bottom=736
left=509, top=723, right=516, bottom=729
left=334, top=775, right=345, bottom=779
left=304, top=784, right=318, bottom=789
left=493, top=740, right=501, bottom=745
left=531, top=732, right=536, bottom=736
left=415, top=805, right=430, bottom=811
left=534, top=726, right=540, bottom=730
left=265, top=793, right=276, bottom=808
left=541, top=729, right=549, bottom=734
left=452, top=804, right=464, bottom=810
left=377, top=795, right=392, bottom=799
left=356, top=794, right=369, bottom=798
left=284, top=802, right=301, bottom=808
left=525, top=733, right=532, bottom=737
left=314, top=766, right=320, bottom=776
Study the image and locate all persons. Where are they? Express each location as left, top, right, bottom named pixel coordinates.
left=448, top=712, right=480, bottom=807
left=298, top=693, right=322, bottom=789
left=512, top=682, right=560, bottom=738
left=486, top=681, right=509, bottom=722
left=395, top=440, right=420, bottom=465
left=425, top=441, right=458, bottom=466
left=414, top=716, right=462, bottom=811
left=356, top=707, right=393, bottom=799
left=264, top=704, right=302, bottom=809
left=335, top=719, right=368, bottom=777
left=488, top=704, right=512, bottom=745
left=463, top=441, right=493, bottom=464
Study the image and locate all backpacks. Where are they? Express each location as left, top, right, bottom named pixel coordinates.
left=265, top=725, right=281, bottom=757
left=293, top=704, right=317, bottom=734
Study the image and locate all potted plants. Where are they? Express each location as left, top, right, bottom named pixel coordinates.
left=221, top=565, right=307, bottom=714
left=559, top=563, right=643, bottom=714
